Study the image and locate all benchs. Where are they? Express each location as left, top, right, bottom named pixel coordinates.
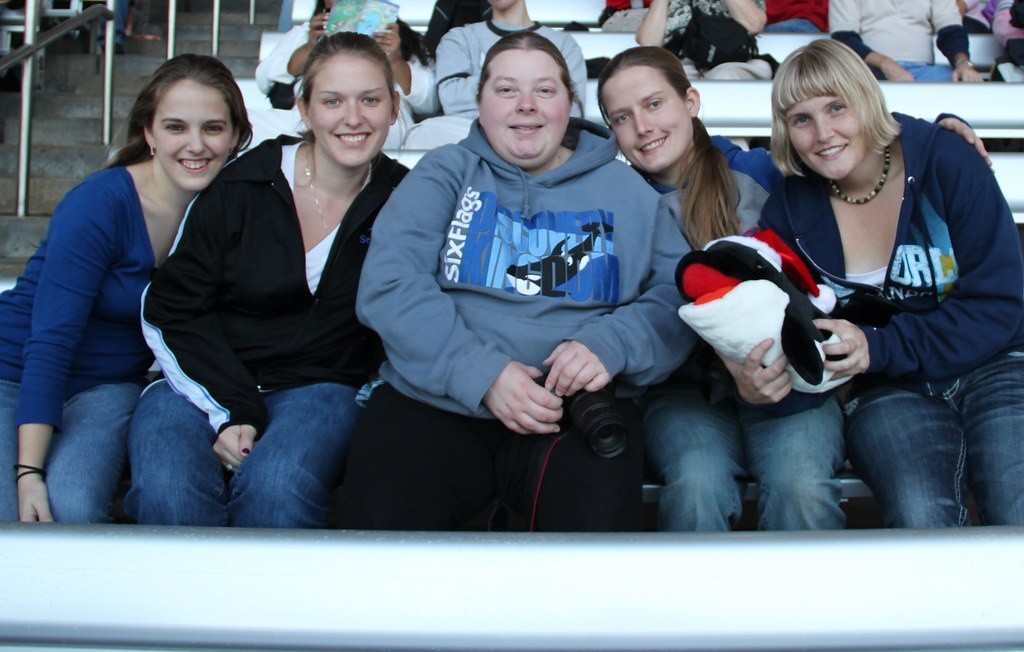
left=232, top=0, right=1024, bottom=224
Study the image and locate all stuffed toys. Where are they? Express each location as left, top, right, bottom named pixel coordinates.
left=675, top=227, right=858, bottom=392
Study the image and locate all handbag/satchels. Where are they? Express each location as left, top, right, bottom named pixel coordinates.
left=665, top=6, right=758, bottom=70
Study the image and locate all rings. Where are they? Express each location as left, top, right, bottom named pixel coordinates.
left=227, top=464, right=232, bottom=471
left=386, top=40, right=391, bottom=45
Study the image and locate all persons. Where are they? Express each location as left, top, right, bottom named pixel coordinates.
left=322, top=34, right=693, bottom=532
left=125, top=29, right=414, bottom=528
left=0, top=53, right=252, bottom=523
left=714, top=38, right=1024, bottom=528
left=597, top=47, right=997, bottom=532
left=0, top=0, right=1024, bottom=118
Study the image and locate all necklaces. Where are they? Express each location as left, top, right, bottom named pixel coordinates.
left=830, top=145, right=890, bottom=204
left=304, top=166, right=327, bottom=228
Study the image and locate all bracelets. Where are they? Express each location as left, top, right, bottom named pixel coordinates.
left=13, top=464, right=46, bottom=481
left=955, top=59, right=974, bottom=66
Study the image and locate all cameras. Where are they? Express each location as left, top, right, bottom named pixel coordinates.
left=534, top=371, right=628, bottom=459
